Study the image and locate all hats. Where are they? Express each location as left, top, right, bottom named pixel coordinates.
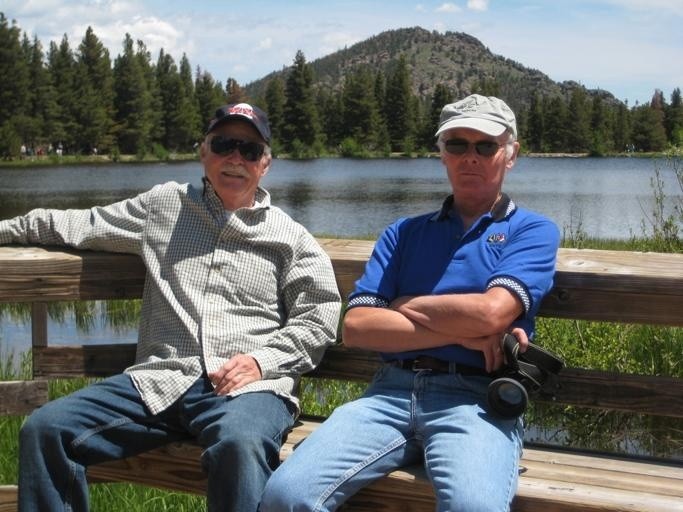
left=206, top=103, right=271, bottom=145
left=434, top=94, right=517, bottom=141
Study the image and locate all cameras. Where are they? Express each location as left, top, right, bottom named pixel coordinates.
left=487, top=333, right=564, bottom=417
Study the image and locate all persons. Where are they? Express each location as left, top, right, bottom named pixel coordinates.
left=14, top=140, right=64, bottom=160
left=0, top=100, right=345, bottom=512
left=257, top=94, right=562, bottom=512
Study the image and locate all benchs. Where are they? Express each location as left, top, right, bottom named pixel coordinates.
left=0, top=238, right=682, bottom=512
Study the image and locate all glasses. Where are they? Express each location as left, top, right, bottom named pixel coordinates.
left=206, top=135, right=265, bottom=162
left=441, top=137, right=506, bottom=158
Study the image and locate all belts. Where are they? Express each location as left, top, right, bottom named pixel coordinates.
left=390, top=357, right=481, bottom=377
